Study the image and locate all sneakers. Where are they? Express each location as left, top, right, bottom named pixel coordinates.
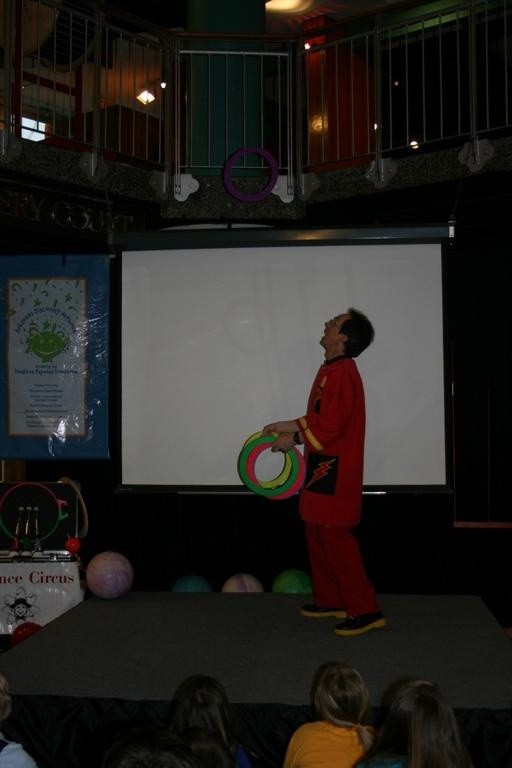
left=299, top=604, right=388, bottom=637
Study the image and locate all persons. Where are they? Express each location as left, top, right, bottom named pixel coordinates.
left=104, top=662, right=476, bottom=766
left=2, top=675, right=37, bottom=767
left=263, top=308, right=386, bottom=637
